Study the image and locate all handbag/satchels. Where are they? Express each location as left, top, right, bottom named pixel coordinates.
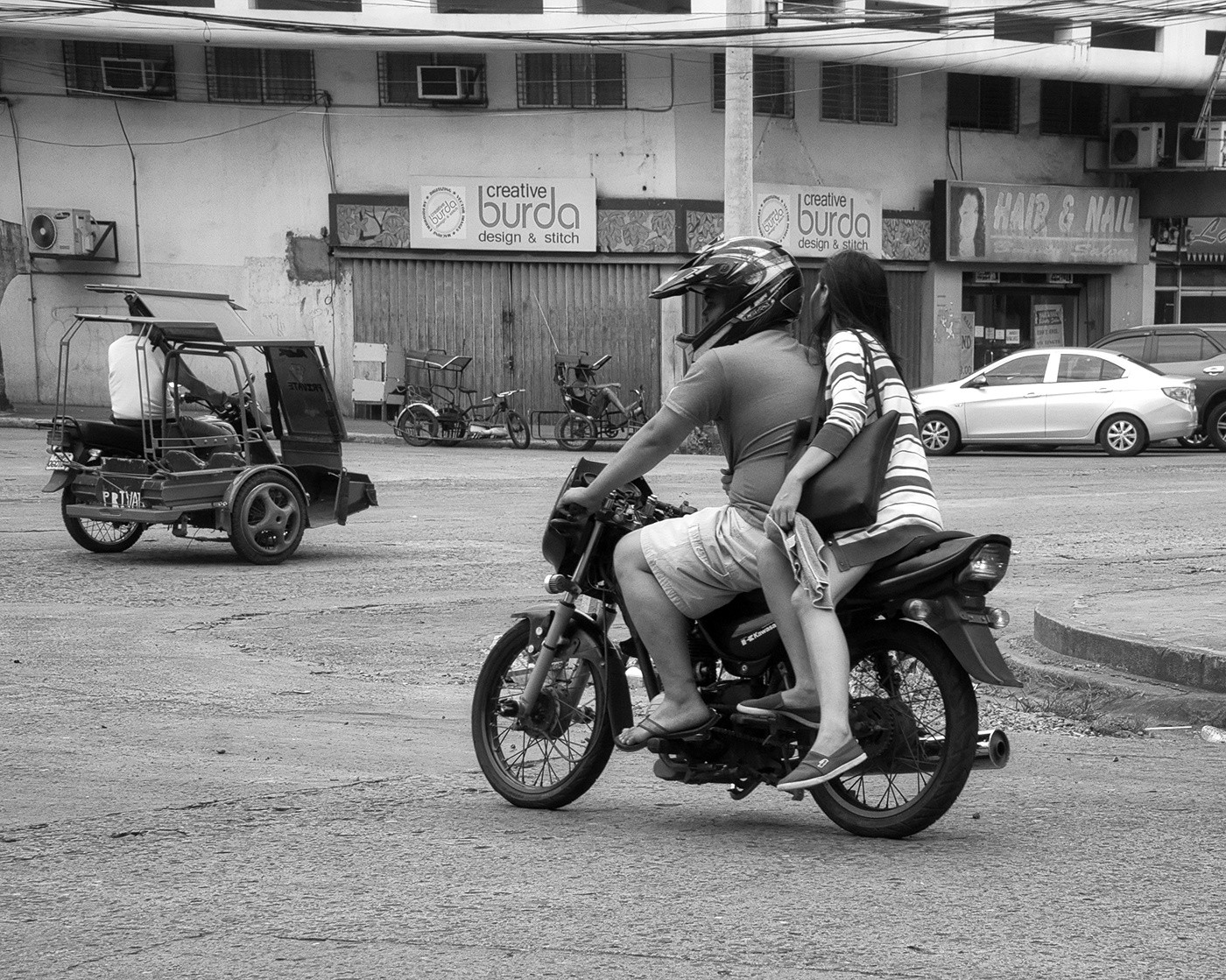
left=784, top=328, right=902, bottom=538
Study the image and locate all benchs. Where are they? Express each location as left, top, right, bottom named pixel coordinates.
left=165, top=449, right=246, bottom=471
left=406, top=386, right=459, bottom=422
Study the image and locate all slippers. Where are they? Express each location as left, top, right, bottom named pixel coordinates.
left=614, top=707, right=722, bottom=752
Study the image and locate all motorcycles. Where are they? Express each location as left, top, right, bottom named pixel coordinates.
left=42, top=283, right=379, bottom=566
left=471, top=457, right=1011, bottom=839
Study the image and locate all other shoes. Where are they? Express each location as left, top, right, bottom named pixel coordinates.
left=623, top=407, right=642, bottom=420
left=776, top=738, right=868, bottom=792
left=735, top=693, right=821, bottom=729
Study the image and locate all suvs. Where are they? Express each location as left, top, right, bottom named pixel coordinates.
left=1069, top=324, right=1226, bottom=451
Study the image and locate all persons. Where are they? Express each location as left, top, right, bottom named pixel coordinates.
left=108, top=306, right=233, bottom=453
left=557, top=235, right=823, bottom=752
left=737, top=249, right=944, bottom=790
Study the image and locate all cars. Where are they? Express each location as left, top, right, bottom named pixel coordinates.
left=909, top=347, right=1198, bottom=457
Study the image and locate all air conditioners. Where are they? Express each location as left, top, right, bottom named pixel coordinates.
left=101, top=55, right=174, bottom=94
left=1176, top=121, right=1226, bottom=168
left=1109, top=122, right=1166, bottom=168
left=26, top=208, right=95, bottom=256
left=416, top=66, right=481, bottom=102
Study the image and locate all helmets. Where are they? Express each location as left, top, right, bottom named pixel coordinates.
left=648, top=236, right=806, bottom=366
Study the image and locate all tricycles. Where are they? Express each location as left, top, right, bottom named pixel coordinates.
left=529, top=350, right=652, bottom=452
left=385, top=348, right=531, bottom=449
